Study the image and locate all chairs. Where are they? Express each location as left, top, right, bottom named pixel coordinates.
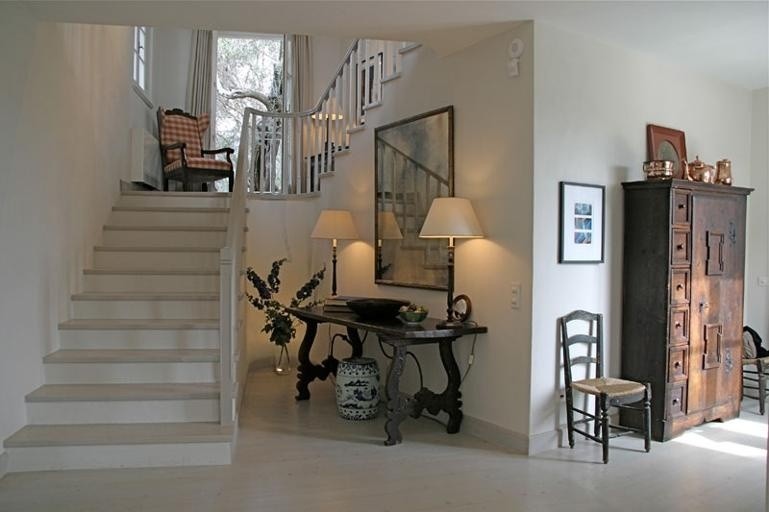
left=561, top=310, right=652, bottom=464
left=741, top=326, right=769, bottom=415
left=157, top=105, right=235, bottom=192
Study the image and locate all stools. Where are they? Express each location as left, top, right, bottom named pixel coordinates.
left=334, top=358, right=380, bottom=420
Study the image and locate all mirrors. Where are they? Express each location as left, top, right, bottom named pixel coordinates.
left=375, top=104, right=454, bottom=291
left=648, top=124, right=687, bottom=180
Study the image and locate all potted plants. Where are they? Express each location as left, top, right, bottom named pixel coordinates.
left=246, top=258, right=326, bottom=374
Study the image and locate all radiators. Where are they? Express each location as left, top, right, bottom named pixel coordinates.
left=130, top=128, right=162, bottom=189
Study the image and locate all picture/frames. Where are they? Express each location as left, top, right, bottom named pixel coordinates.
left=356, top=52, right=383, bottom=126
left=559, top=181, right=606, bottom=264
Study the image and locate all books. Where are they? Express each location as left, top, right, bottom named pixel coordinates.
left=324, top=295, right=364, bottom=305
left=323, top=304, right=354, bottom=313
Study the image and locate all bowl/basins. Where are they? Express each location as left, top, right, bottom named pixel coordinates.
left=347, top=298, right=429, bottom=323
left=642, top=160, right=675, bottom=181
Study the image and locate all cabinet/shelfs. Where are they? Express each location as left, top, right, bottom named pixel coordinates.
left=618, top=178, right=755, bottom=444
left=305, top=146, right=350, bottom=192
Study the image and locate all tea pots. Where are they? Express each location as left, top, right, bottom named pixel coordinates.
left=682, top=155, right=716, bottom=186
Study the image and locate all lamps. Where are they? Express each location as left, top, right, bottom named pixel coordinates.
left=377, top=211, right=403, bottom=278
left=310, top=210, right=360, bottom=300
left=418, top=198, right=486, bottom=327
left=312, top=97, right=343, bottom=151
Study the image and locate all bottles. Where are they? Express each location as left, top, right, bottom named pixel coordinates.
left=717, top=157, right=733, bottom=186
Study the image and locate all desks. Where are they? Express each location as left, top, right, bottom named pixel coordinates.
left=285, top=299, right=488, bottom=446
